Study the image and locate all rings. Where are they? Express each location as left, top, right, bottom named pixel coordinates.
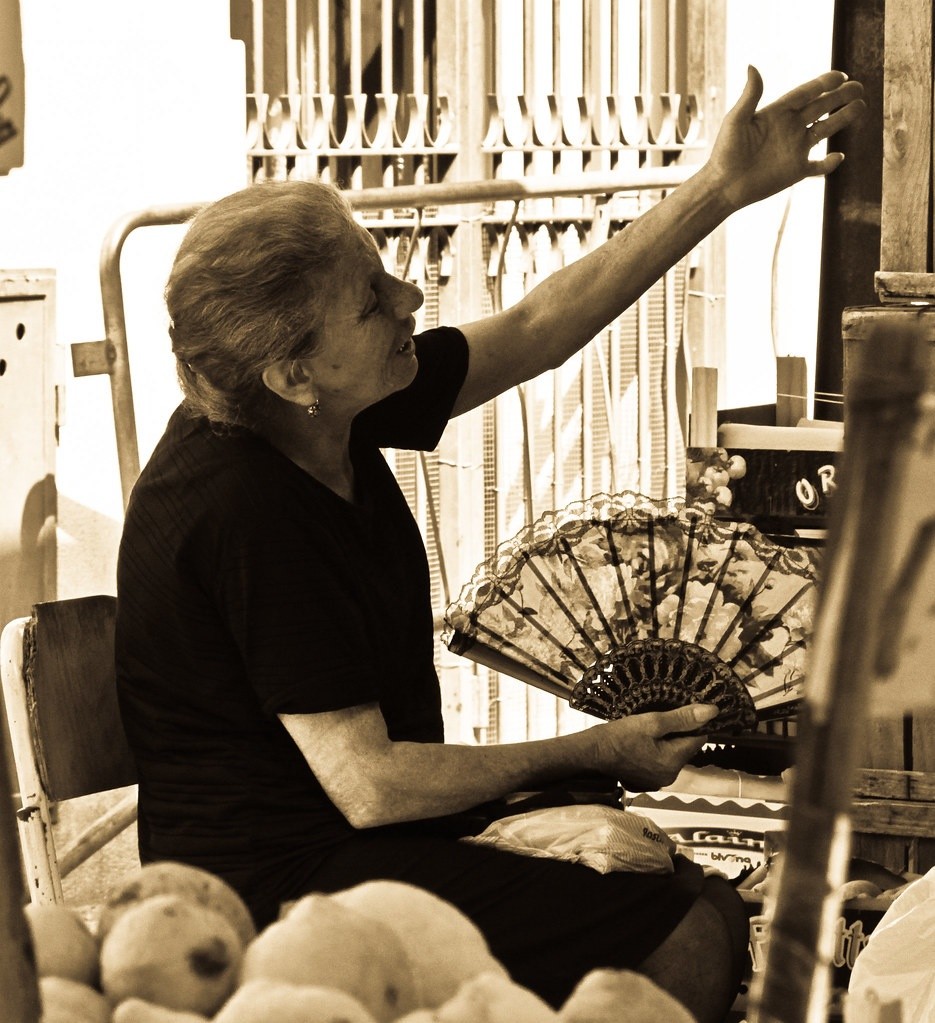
left=808, top=126, right=821, bottom=144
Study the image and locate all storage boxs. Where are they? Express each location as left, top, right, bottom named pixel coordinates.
left=685, top=357, right=908, bottom=802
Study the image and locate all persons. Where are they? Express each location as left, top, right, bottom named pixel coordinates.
left=111, top=63, right=866, bottom=1023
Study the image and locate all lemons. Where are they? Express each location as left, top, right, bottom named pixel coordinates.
left=20, top=857, right=696, bottom=1023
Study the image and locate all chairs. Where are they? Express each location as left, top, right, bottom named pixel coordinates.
left=1, top=596, right=144, bottom=909
